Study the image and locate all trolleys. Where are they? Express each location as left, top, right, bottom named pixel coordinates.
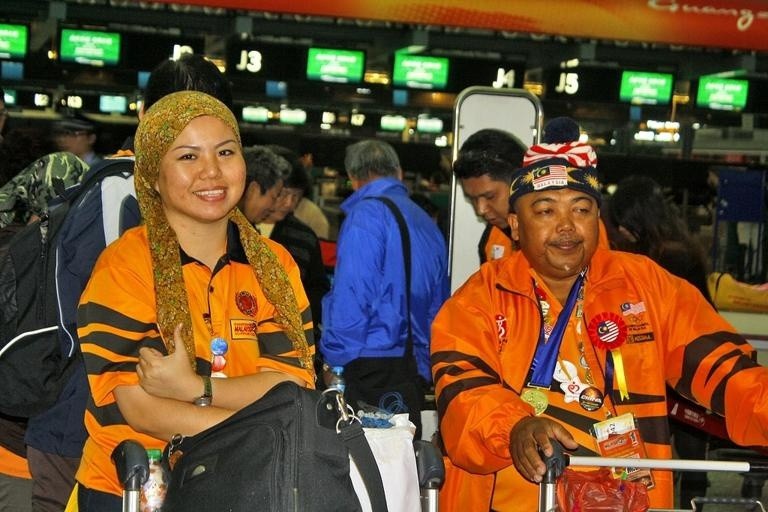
left=534, top=437, right=768, bottom=511
left=111, top=441, right=444, bottom=511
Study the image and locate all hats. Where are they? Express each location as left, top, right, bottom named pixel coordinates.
left=50, top=102, right=101, bottom=136
left=506, top=114, right=604, bottom=209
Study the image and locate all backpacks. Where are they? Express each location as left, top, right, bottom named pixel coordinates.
left=0, top=159, right=136, bottom=422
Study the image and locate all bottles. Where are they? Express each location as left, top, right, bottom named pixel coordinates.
left=140, top=448, right=172, bottom=511
left=328, top=364, right=345, bottom=395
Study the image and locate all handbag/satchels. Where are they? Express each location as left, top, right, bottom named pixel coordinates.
left=341, top=356, right=429, bottom=447
left=156, top=380, right=389, bottom=512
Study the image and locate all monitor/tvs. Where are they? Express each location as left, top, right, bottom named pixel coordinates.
left=393, top=53, right=449, bottom=90
left=61, top=28, right=120, bottom=66
left=620, top=67, right=674, bottom=106
left=696, top=73, right=750, bottom=112
left=307, top=47, right=366, bottom=86
left=0, top=22, right=28, bottom=62
